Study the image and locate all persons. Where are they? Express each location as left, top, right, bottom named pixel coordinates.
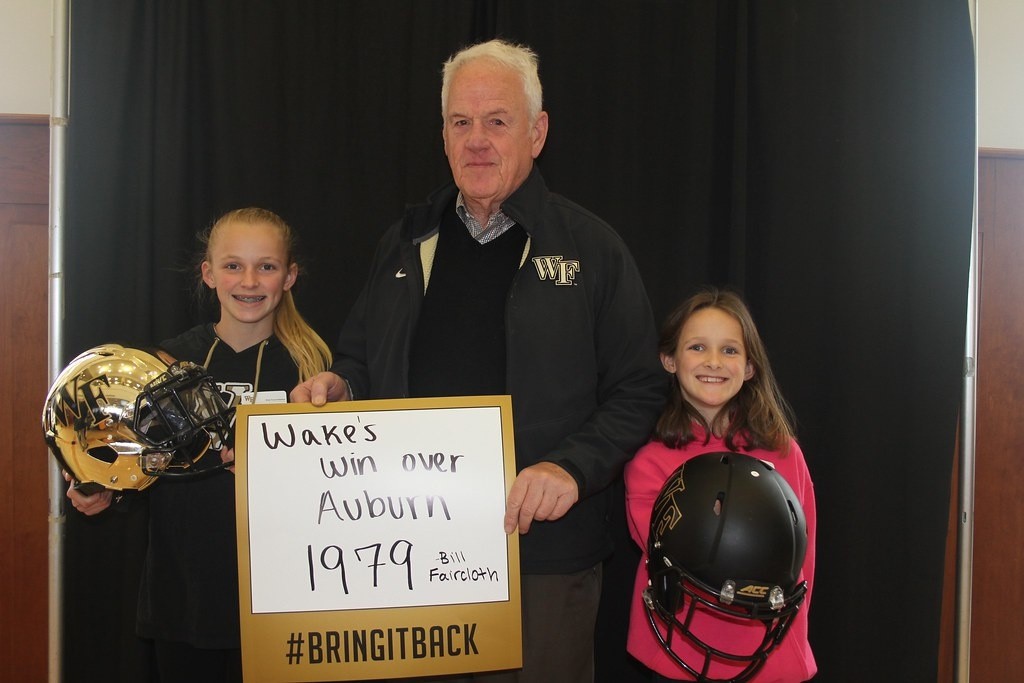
left=62, top=203, right=332, bottom=683
left=281, top=36, right=644, bottom=681
left=622, top=287, right=821, bottom=683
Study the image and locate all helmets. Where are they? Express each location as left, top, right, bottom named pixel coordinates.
left=645, top=452, right=807, bottom=627
left=42, top=344, right=213, bottom=495
